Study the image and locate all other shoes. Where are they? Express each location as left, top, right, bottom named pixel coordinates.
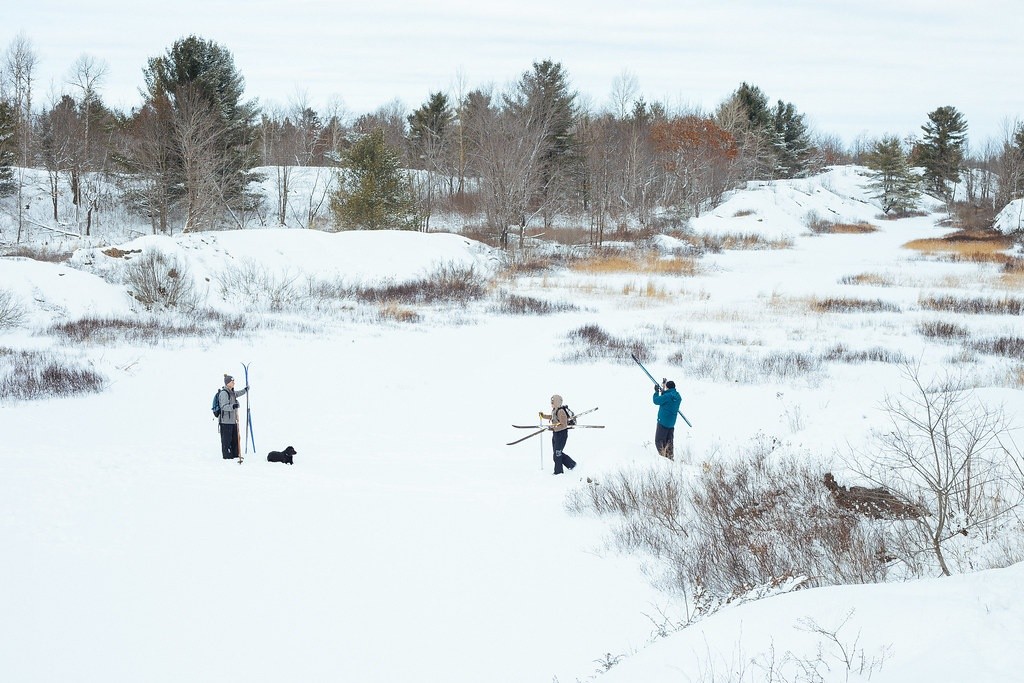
left=568, top=462, right=576, bottom=470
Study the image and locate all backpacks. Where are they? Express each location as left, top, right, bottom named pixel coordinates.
left=556, top=405, right=577, bottom=425
left=211, top=389, right=230, bottom=417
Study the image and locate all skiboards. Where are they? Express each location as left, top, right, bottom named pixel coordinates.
left=630, top=353, right=692, bottom=429
left=507, top=407, right=605, bottom=446
left=241, top=361, right=257, bottom=454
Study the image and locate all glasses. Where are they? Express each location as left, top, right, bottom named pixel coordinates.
left=654, top=384, right=659, bottom=391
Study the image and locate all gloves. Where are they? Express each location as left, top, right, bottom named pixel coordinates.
left=233, top=403, right=240, bottom=409
left=548, top=426, right=554, bottom=431
left=539, top=412, right=544, bottom=418
left=245, top=385, right=250, bottom=391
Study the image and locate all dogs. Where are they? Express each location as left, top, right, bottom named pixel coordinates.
left=267, top=446, right=297, bottom=465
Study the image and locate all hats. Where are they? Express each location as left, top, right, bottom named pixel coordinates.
left=666, top=381, right=675, bottom=389
left=224, top=374, right=234, bottom=386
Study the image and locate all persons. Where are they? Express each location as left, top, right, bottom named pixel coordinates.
left=539, top=394, right=577, bottom=475
left=218, top=375, right=250, bottom=459
left=652, top=377, right=682, bottom=462
